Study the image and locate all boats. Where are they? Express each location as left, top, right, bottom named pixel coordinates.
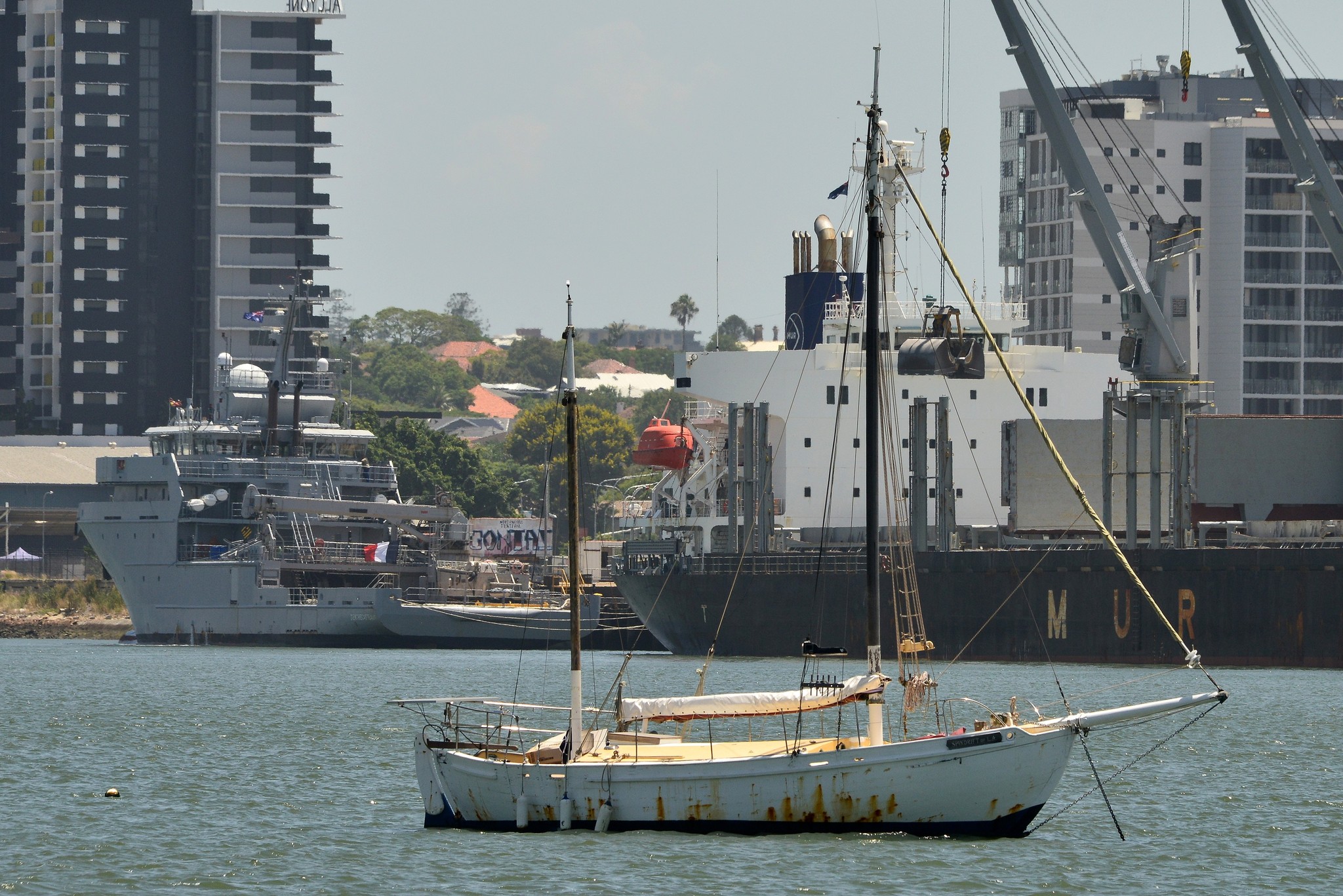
left=630, top=399, right=693, bottom=471
left=610, top=0, right=1343, bottom=672
left=74, top=260, right=602, bottom=649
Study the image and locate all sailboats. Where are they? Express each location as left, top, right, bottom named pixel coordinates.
left=388, top=40, right=1232, bottom=840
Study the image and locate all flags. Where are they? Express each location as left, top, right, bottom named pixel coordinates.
left=170, top=398, right=182, bottom=407
left=244, top=311, right=264, bottom=323
left=827, top=181, right=848, bottom=200
left=364, top=540, right=399, bottom=565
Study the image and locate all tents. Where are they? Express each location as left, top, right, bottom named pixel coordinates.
left=0, top=547, right=43, bottom=561
